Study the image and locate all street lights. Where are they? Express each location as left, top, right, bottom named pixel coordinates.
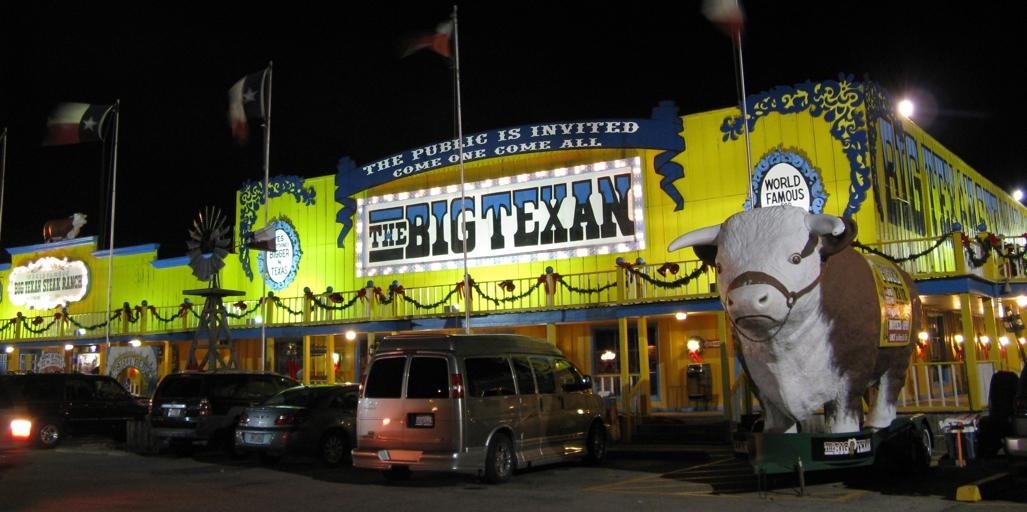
left=1002, top=305, right=1027, bottom=365
left=600, top=349, right=618, bottom=374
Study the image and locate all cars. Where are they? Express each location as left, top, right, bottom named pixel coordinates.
left=233, top=381, right=360, bottom=469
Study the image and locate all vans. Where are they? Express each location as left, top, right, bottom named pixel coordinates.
left=346, top=330, right=610, bottom=486
left=0, top=371, right=145, bottom=450
left=145, top=373, right=301, bottom=459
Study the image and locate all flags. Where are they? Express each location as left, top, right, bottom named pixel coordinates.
left=395, top=15, right=455, bottom=72
left=245, top=222, right=278, bottom=254
left=702, top=0, right=745, bottom=60
left=227, top=64, right=268, bottom=145
left=39, top=98, right=116, bottom=149
